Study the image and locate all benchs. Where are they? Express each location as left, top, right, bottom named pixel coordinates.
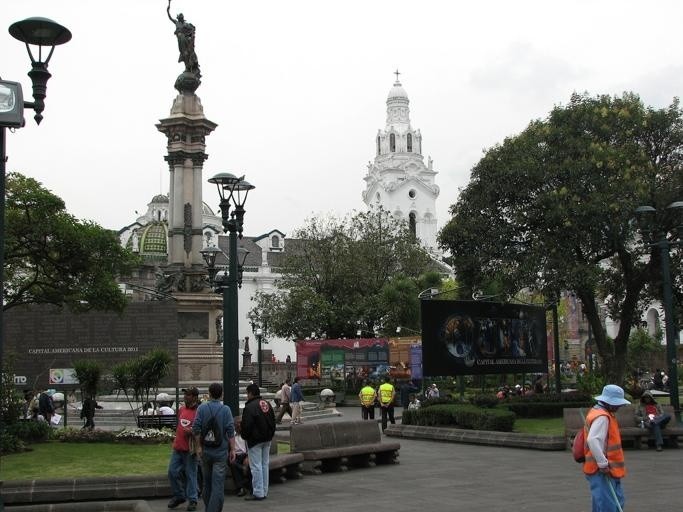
left=137, top=415, right=179, bottom=431
left=290, top=419, right=401, bottom=475
left=236, top=436, right=304, bottom=484
left=563, top=406, right=683, bottom=453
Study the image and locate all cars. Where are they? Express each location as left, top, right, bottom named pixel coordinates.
left=496, top=385, right=547, bottom=400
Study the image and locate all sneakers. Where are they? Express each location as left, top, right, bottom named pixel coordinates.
left=168, top=488, right=266, bottom=512
left=656, top=445, right=662, bottom=451
left=645, top=422, right=653, bottom=428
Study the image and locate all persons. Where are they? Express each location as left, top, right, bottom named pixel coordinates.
left=495, top=359, right=597, bottom=401
left=407, top=394, right=422, bottom=410
left=358, top=380, right=377, bottom=420
left=572, top=384, right=632, bottom=512
left=424, top=383, right=440, bottom=399
left=654, top=368, right=664, bottom=391
left=39, top=393, right=55, bottom=426
left=240, top=384, right=276, bottom=501
left=227, top=416, right=254, bottom=498
left=191, top=383, right=236, bottom=512
left=139, top=400, right=175, bottom=416
left=377, top=377, right=396, bottom=434
left=164, top=0, right=202, bottom=76
left=80, top=393, right=103, bottom=430
left=167, top=386, right=200, bottom=512
left=274, top=376, right=306, bottom=425
left=633, top=389, right=671, bottom=452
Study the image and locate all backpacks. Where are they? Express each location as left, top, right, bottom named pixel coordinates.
left=202, top=402, right=224, bottom=447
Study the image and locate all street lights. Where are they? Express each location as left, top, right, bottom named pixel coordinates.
left=356, top=330, right=383, bottom=338
left=254, top=325, right=264, bottom=389
left=395, top=326, right=421, bottom=336
left=0, top=14, right=71, bottom=307
left=633, top=198, right=683, bottom=423
left=202, top=172, right=253, bottom=418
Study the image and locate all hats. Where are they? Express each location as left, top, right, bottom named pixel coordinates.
left=182, top=386, right=199, bottom=395
left=594, top=384, right=631, bottom=406
